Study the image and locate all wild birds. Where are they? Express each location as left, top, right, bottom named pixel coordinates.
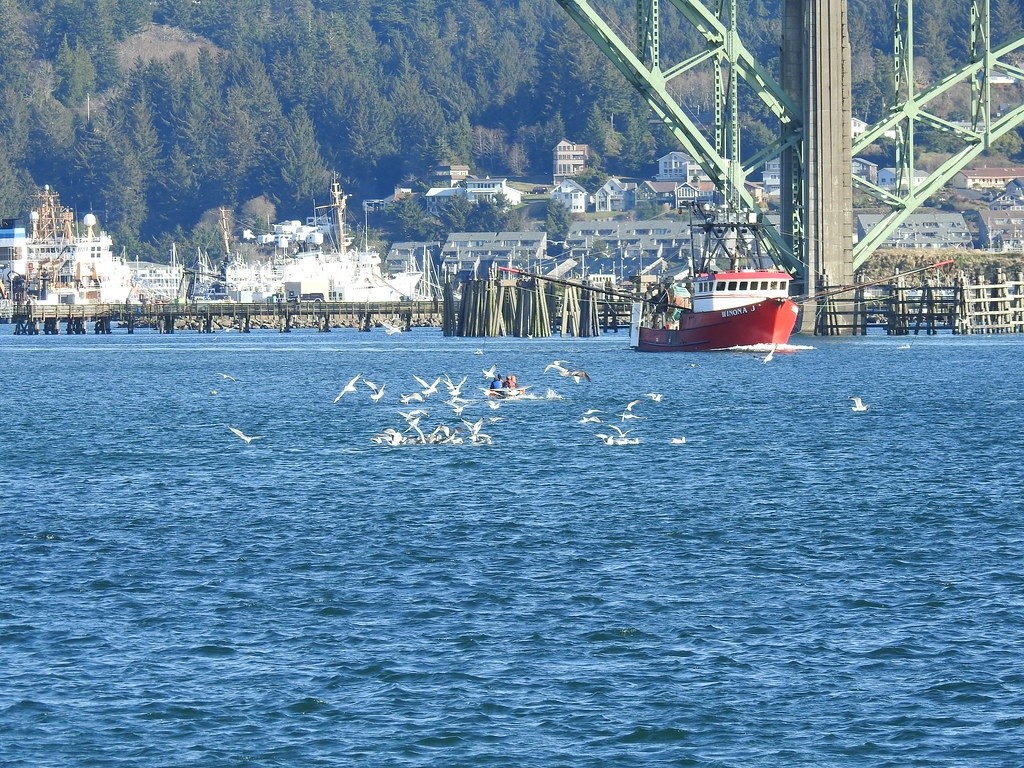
left=332, top=370, right=365, bottom=404
left=506, top=388, right=563, bottom=401
left=615, top=400, right=646, bottom=422
left=442, top=372, right=474, bottom=416
left=479, top=363, right=505, bottom=410
left=473, top=348, right=483, bottom=355
left=670, top=436, right=686, bottom=444
left=897, top=343, right=911, bottom=349
left=369, top=416, right=507, bottom=447
left=690, top=363, right=696, bottom=367
left=849, top=397, right=869, bottom=412
left=399, top=392, right=426, bottom=406
left=379, top=319, right=406, bottom=335
left=362, top=378, right=388, bottom=403
left=760, top=343, right=778, bottom=364
left=226, top=425, right=265, bottom=444
left=216, top=372, right=236, bottom=382
left=527, top=333, right=534, bottom=340
left=593, top=424, right=640, bottom=446
left=579, top=408, right=608, bottom=424
left=644, top=393, right=663, bottom=402
left=544, top=360, right=591, bottom=384
left=411, top=373, right=441, bottom=398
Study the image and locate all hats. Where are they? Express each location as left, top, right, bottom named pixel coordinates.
left=498, top=374, right=501, bottom=379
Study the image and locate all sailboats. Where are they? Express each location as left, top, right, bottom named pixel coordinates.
left=497, top=128, right=954, bottom=352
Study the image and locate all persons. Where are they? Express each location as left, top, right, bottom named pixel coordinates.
left=490, top=374, right=517, bottom=389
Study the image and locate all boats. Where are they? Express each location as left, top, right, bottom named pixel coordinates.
left=489, top=386, right=530, bottom=399
left=2, top=170, right=423, bottom=324
